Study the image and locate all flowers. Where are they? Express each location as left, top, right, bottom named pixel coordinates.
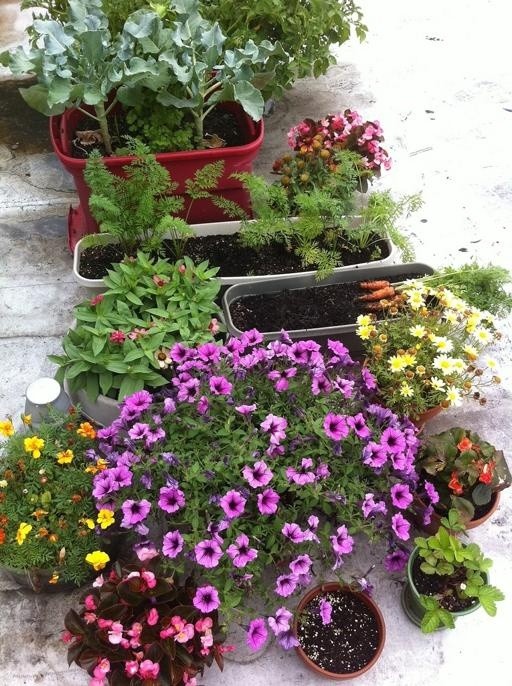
left=352, top=302, right=499, bottom=393
left=276, top=106, right=394, bottom=185
left=73, top=585, right=226, bottom=683
left=0, top=415, right=104, bottom=559
left=56, top=262, right=240, bottom=373
left=102, top=334, right=409, bottom=586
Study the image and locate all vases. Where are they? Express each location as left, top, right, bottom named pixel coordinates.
left=396, top=399, right=446, bottom=427
left=217, top=260, right=440, bottom=338
left=63, top=370, right=166, bottom=427
left=8, top=567, right=55, bottom=592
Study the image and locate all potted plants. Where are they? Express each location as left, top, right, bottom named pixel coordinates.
left=411, top=433, right=504, bottom=532
left=16, top=4, right=279, bottom=233
left=72, top=139, right=408, bottom=282
left=401, top=518, right=499, bottom=634
left=289, top=584, right=386, bottom=678
